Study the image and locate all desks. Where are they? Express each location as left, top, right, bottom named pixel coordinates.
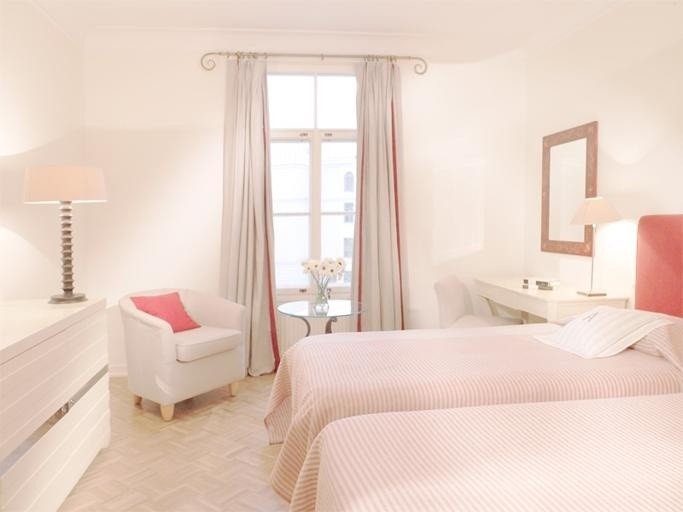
left=275, top=300, right=366, bottom=337
left=476, top=277, right=633, bottom=327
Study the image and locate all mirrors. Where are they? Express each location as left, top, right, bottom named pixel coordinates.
left=539, top=120, right=598, bottom=257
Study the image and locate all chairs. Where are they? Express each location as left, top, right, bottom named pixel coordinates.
left=117, top=287, right=251, bottom=423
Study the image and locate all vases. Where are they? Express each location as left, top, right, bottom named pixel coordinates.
left=314, top=285, right=328, bottom=316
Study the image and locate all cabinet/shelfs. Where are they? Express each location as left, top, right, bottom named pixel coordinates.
left=1, top=300, right=113, bottom=512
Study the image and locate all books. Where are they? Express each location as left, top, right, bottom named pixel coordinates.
left=523, top=278, right=561, bottom=285
left=521, top=284, right=564, bottom=290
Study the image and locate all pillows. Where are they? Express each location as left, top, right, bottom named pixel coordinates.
left=629, top=311, right=681, bottom=370
left=130, top=291, right=202, bottom=334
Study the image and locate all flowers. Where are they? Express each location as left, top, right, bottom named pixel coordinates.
left=300, top=257, right=347, bottom=289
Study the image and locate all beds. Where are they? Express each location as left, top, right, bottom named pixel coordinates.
left=264, top=211, right=683, bottom=504
left=286, top=391, right=682, bottom=511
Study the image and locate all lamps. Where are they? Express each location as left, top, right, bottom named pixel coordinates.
left=19, top=161, right=114, bottom=304
left=568, top=195, right=624, bottom=299
